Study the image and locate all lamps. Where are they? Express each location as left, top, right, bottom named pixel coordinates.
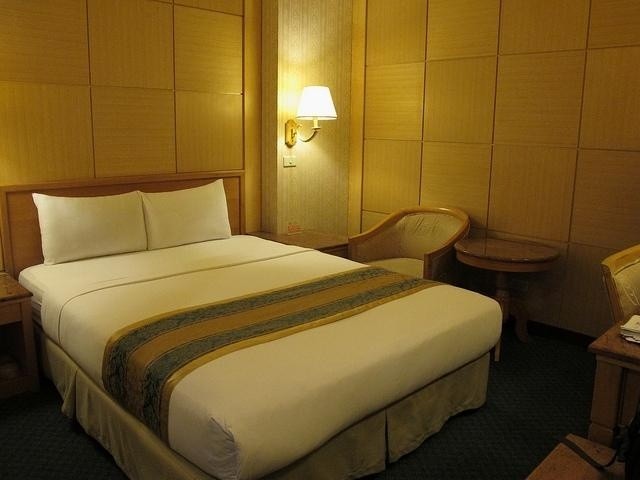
left=285, top=85, right=338, bottom=147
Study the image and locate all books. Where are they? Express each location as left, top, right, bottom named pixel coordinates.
left=620, top=314, right=640, bottom=344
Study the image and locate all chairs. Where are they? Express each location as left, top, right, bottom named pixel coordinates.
left=601, top=244, right=640, bottom=325
left=348, top=205, right=471, bottom=282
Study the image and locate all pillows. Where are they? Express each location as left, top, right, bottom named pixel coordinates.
left=137, top=178, right=231, bottom=251
left=32, top=191, right=148, bottom=265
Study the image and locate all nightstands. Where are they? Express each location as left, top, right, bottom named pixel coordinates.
left=0, top=270, right=39, bottom=402
left=246, top=228, right=351, bottom=259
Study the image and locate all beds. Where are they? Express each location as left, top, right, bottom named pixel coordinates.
left=1, top=171, right=504, bottom=479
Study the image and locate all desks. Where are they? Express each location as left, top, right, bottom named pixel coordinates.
left=524, top=431, right=626, bottom=479
left=587, top=305, right=640, bottom=450
left=454, top=236, right=561, bottom=362
